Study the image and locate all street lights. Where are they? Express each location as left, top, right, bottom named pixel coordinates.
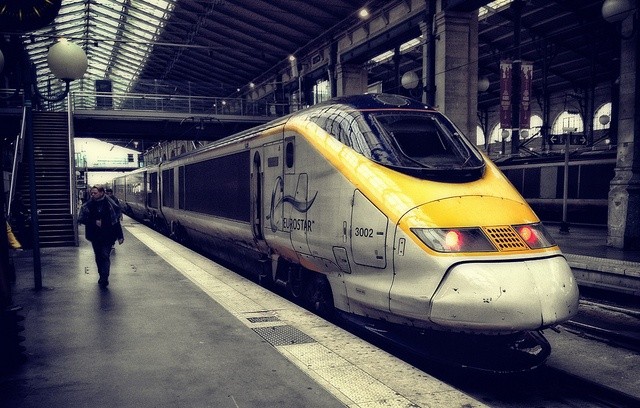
left=401, top=70, right=436, bottom=106
left=500, top=130, right=512, bottom=155
left=519, top=130, right=530, bottom=153
left=1, top=41, right=88, bottom=292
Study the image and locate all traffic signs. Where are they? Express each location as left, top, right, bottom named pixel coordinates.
left=570, top=135, right=587, bottom=145
left=549, top=134, right=565, bottom=145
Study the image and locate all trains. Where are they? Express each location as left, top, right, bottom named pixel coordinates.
left=102, top=93, right=580, bottom=374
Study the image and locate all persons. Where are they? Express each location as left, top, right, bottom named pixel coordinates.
left=81, top=184, right=125, bottom=287
left=98, top=187, right=105, bottom=193
left=105, top=189, right=119, bottom=205
